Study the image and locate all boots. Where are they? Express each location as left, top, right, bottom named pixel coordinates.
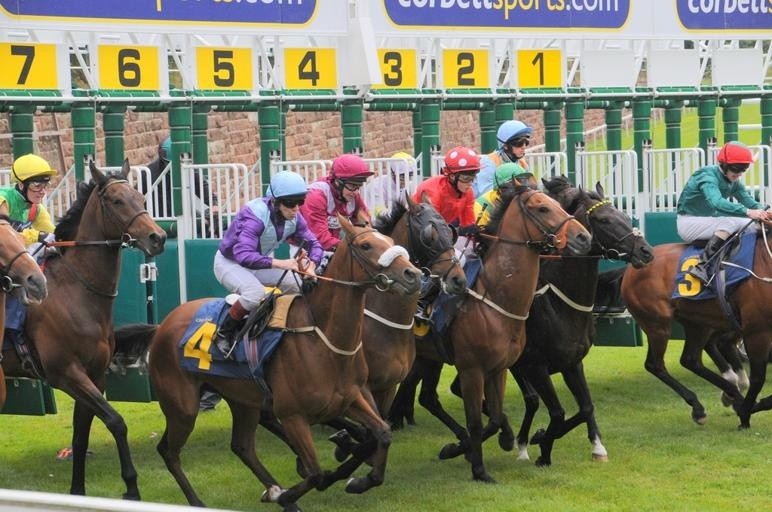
left=690, top=234, right=726, bottom=290
left=414, top=281, right=439, bottom=326
left=212, top=312, right=240, bottom=362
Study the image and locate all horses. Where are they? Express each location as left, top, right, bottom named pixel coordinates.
left=0, top=156, right=169, bottom=501
left=595, top=226, right=772, bottom=432
left=415, top=172, right=594, bottom=485
left=257, top=189, right=469, bottom=493
left=0, top=213, right=50, bottom=411
left=112, top=206, right=422, bottom=512
left=703, top=337, right=751, bottom=408
left=540, top=174, right=586, bottom=213
left=417, top=179, right=657, bottom=470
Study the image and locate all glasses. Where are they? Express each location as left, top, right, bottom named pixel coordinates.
left=338, top=180, right=362, bottom=191
left=30, top=181, right=51, bottom=190
left=458, top=173, right=476, bottom=182
left=729, top=164, right=746, bottom=173
left=507, top=138, right=529, bottom=146
left=280, top=196, right=304, bottom=208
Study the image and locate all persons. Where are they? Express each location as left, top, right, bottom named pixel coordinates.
left=411, top=146, right=483, bottom=328
left=673, top=138, right=771, bottom=291
left=0, top=153, right=58, bottom=345
left=213, top=170, right=324, bottom=363
left=471, top=114, right=534, bottom=201
left=471, top=162, right=534, bottom=232
left=301, top=152, right=376, bottom=255
left=359, top=153, right=424, bottom=228
left=138, top=135, right=220, bottom=225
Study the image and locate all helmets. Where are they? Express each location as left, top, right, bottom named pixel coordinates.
left=9, top=155, right=57, bottom=182
left=718, top=141, right=755, bottom=164
left=265, top=170, right=308, bottom=198
left=332, top=154, right=375, bottom=178
left=443, top=146, right=485, bottom=174
left=390, top=152, right=417, bottom=175
left=497, top=120, right=533, bottom=150
left=493, top=163, right=534, bottom=191
left=159, top=136, right=173, bottom=162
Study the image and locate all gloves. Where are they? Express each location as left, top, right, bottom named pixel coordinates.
left=458, top=224, right=481, bottom=237
left=38, top=230, right=56, bottom=243
